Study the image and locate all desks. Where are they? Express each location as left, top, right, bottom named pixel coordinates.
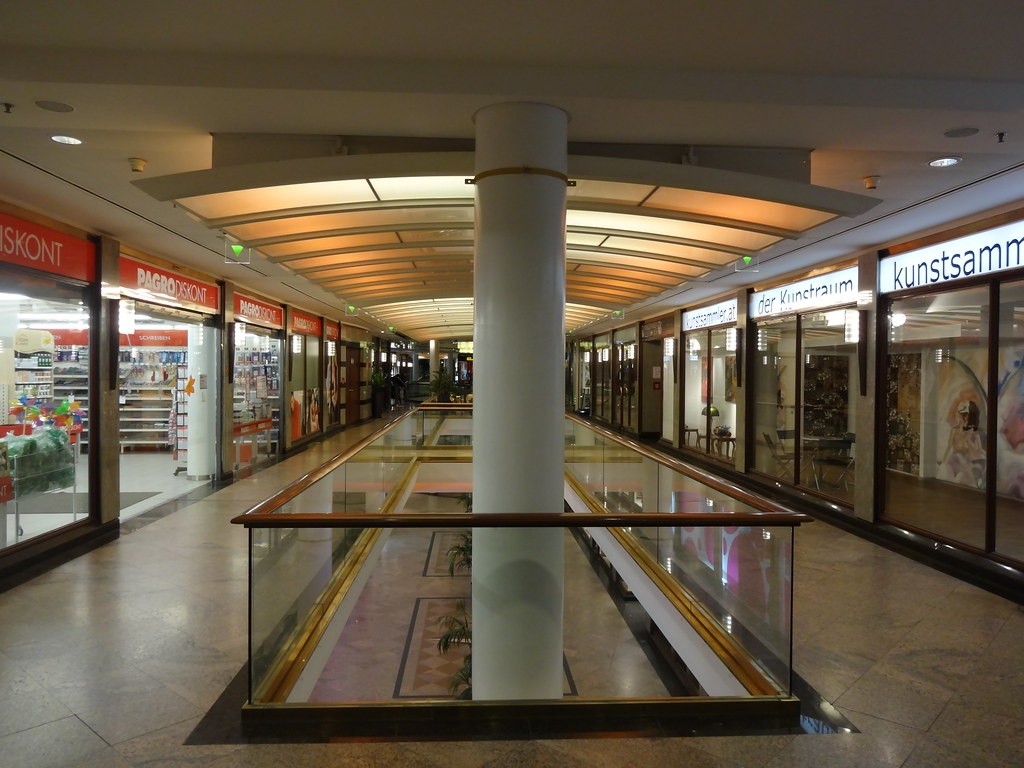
left=685, top=428, right=701, bottom=450
left=696, top=434, right=736, bottom=460
left=800, top=436, right=843, bottom=486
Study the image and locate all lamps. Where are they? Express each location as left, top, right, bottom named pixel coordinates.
left=703, top=406, right=719, bottom=435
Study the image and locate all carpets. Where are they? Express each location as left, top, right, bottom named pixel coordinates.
left=5, top=490, right=163, bottom=514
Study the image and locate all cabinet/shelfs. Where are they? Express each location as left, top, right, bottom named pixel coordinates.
left=230, top=344, right=278, bottom=462
left=0, top=330, right=190, bottom=479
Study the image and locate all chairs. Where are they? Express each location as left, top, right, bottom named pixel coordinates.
left=763, top=429, right=855, bottom=494
